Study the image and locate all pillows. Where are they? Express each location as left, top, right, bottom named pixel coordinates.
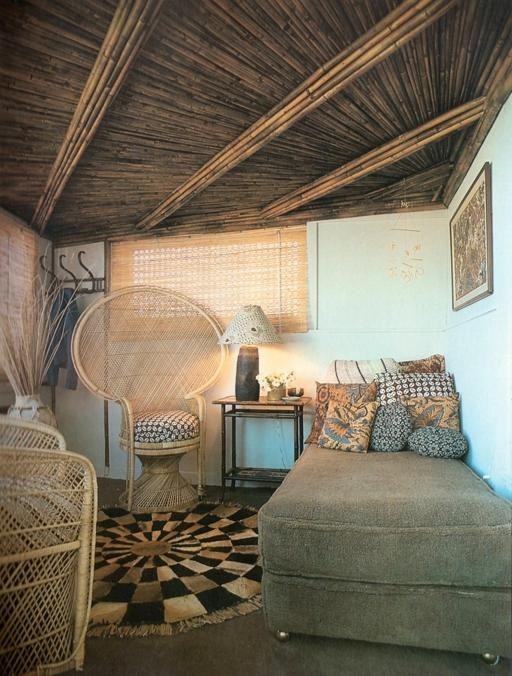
left=304, top=354, right=469, bottom=460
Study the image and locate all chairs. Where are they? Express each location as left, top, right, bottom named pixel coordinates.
left=68, top=283, right=229, bottom=512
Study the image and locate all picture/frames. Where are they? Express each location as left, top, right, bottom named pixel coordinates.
left=447, top=161, right=493, bottom=313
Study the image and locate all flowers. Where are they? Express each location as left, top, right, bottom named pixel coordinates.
left=254, top=368, right=295, bottom=392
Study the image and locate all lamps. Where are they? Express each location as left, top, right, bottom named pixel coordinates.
left=216, top=305, right=283, bottom=401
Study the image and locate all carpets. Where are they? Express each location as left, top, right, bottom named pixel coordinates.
left=82, top=500, right=264, bottom=638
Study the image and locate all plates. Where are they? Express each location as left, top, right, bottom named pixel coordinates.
left=281, top=395, right=301, bottom=402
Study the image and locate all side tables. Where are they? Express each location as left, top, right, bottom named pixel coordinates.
left=212, top=395, right=311, bottom=494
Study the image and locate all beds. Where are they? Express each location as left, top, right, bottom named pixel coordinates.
left=257, top=355, right=512, bottom=667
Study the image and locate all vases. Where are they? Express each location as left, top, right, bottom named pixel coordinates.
left=266, top=388, right=286, bottom=401
left=6, top=393, right=58, bottom=430
